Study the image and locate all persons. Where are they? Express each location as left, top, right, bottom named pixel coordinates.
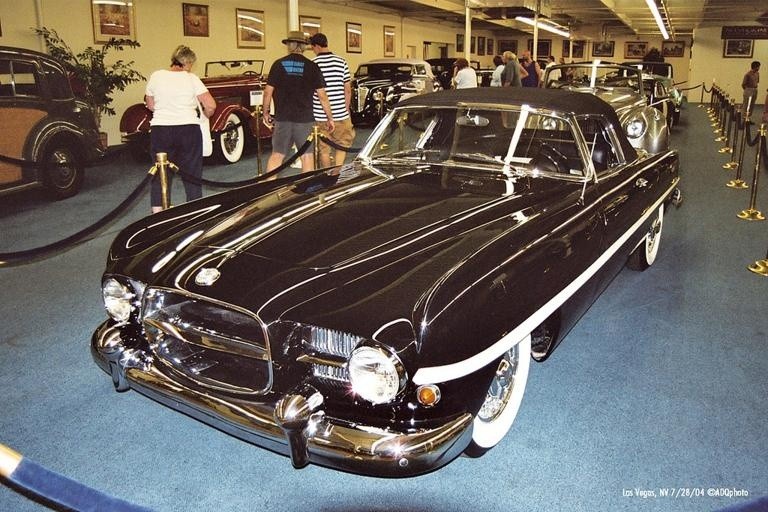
left=452, top=50, right=577, bottom=90
left=261, top=30, right=335, bottom=180
left=309, top=31, right=355, bottom=170
left=145, top=44, right=216, bottom=213
left=742, top=62, right=760, bottom=126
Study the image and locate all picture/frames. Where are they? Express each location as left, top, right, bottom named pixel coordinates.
left=384, top=25, right=396, bottom=57
left=236, top=8, right=265, bottom=49
left=183, top=2, right=209, bottom=37
left=723, top=39, right=754, bottom=59
left=299, top=15, right=321, bottom=50
left=346, top=22, right=362, bottom=53
left=91, top=0, right=138, bottom=45
left=456, top=34, right=685, bottom=58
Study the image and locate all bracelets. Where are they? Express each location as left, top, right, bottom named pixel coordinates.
left=327, top=115, right=333, bottom=120
left=262, top=105, right=270, bottom=113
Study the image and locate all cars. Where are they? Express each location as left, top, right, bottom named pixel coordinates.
left=120, top=59, right=275, bottom=164
left=521, top=61, right=669, bottom=155
left=425, top=58, right=683, bottom=131
left=349, top=59, right=444, bottom=128
left=89, top=87, right=681, bottom=479
left=0, top=45, right=105, bottom=199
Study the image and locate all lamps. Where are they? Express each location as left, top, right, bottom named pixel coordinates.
left=646, top=0, right=670, bottom=40
left=515, top=16, right=570, bottom=38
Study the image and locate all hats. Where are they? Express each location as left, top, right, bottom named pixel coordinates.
left=282, top=31, right=311, bottom=46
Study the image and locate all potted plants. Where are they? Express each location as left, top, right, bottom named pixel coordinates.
left=30, top=27, right=147, bottom=151
